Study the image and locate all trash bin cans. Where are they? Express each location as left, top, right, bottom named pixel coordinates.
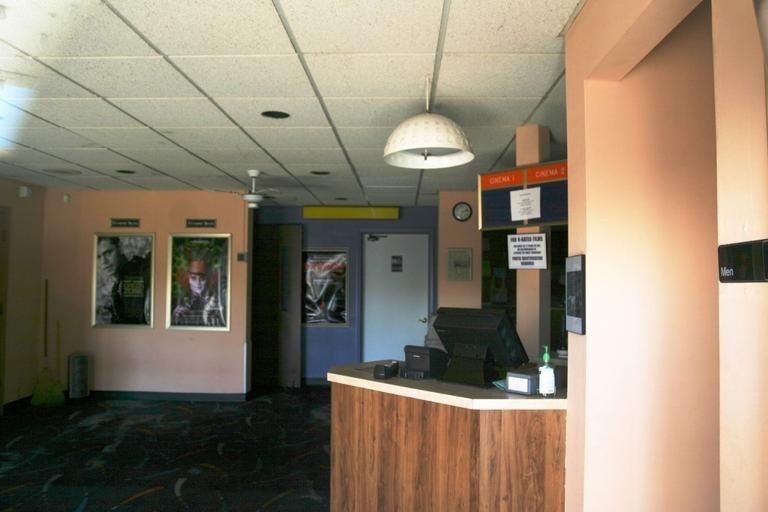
left=67, top=354, right=89, bottom=399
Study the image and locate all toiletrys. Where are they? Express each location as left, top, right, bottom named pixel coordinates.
left=537, top=345, right=558, bottom=397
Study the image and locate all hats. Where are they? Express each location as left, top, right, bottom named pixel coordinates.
left=189, top=261, right=209, bottom=275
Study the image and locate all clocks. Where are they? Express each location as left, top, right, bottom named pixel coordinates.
left=452, top=201, right=473, bottom=222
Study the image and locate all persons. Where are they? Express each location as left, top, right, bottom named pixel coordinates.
left=172, top=260, right=226, bottom=327
left=97, top=237, right=151, bottom=323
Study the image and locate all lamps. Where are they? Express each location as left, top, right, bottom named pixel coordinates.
left=381, top=77, right=477, bottom=170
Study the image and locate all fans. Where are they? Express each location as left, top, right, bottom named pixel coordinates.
left=206, top=168, right=297, bottom=210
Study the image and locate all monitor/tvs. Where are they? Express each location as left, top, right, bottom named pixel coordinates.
left=432, top=307, right=530, bottom=389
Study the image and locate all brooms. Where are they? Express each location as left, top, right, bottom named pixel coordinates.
left=31, top=280, right=66, bottom=404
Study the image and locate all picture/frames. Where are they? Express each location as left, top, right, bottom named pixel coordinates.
left=91, top=231, right=233, bottom=332
left=301, top=249, right=351, bottom=328
left=565, top=254, right=586, bottom=335
